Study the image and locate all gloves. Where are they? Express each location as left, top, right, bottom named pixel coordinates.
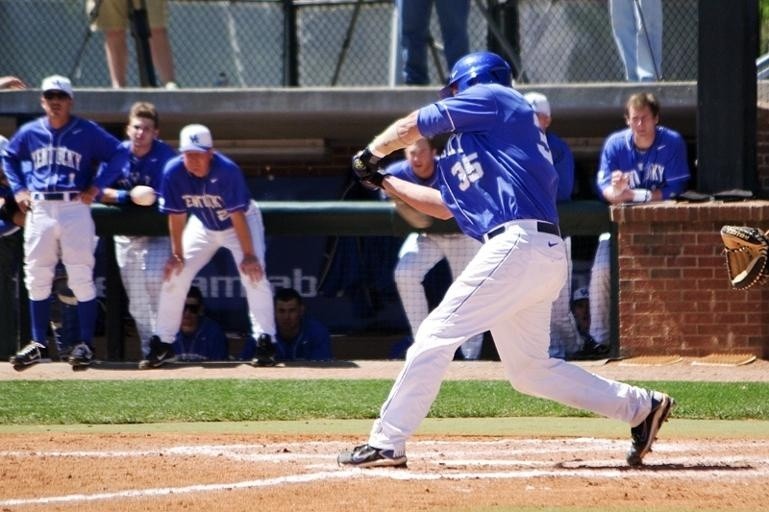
left=353, top=148, right=392, bottom=190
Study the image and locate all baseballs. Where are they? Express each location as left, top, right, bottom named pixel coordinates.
left=132, top=184, right=155, bottom=206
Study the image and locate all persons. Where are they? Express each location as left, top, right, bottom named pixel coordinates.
left=571, top=90, right=691, bottom=361
left=396, top=1, right=472, bottom=85
left=524, top=92, right=587, bottom=360
left=607, top=1, right=663, bottom=82
left=239, top=287, right=333, bottom=362
left=1, top=73, right=130, bottom=366
left=134, top=122, right=278, bottom=369
left=95, top=101, right=178, bottom=359
left=334, top=50, right=676, bottom=469
left=171, top=286, right=228, bottom=362
left=567, top=287, right=618, bottom=361
left=86, top=0, right=182, bottom=89
left=381, top=134, right=485, bottom=360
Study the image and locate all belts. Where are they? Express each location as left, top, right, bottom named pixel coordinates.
left=33, top=193, right=79, bottom=201
left=487, top=221, right=561, bottom=239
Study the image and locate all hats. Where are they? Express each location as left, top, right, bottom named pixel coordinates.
left=178, top=123, right=213, bottom=153
left=41, top=74, right=72, bottom=100
left=522, top=92, right=552, bottom=118
left=572, top=286, right=589, bottom=304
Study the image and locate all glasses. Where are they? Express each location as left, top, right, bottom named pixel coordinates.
left=185, top=303, right=201, bottom=313
left=43, top=92, right=68, bottom=99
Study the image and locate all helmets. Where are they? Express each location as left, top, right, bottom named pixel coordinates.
left=440, top=51, right=512, bottom=95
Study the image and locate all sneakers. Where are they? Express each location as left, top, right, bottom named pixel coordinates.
left=138, top=335, right=177, bottom=370
left=336, top=443, right=408, bottom=469
left=626, top=389, right=677, bottom=465
left=250, top=334, right=278, bottom=367
left=575, top=338, right=609, bottom=356
left=68, top=340, right=96, bottom=366
left=9, top=340, right=51, bottom=366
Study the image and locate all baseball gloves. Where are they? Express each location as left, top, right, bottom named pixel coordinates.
left=719, top=226, right=769, bottom=291
